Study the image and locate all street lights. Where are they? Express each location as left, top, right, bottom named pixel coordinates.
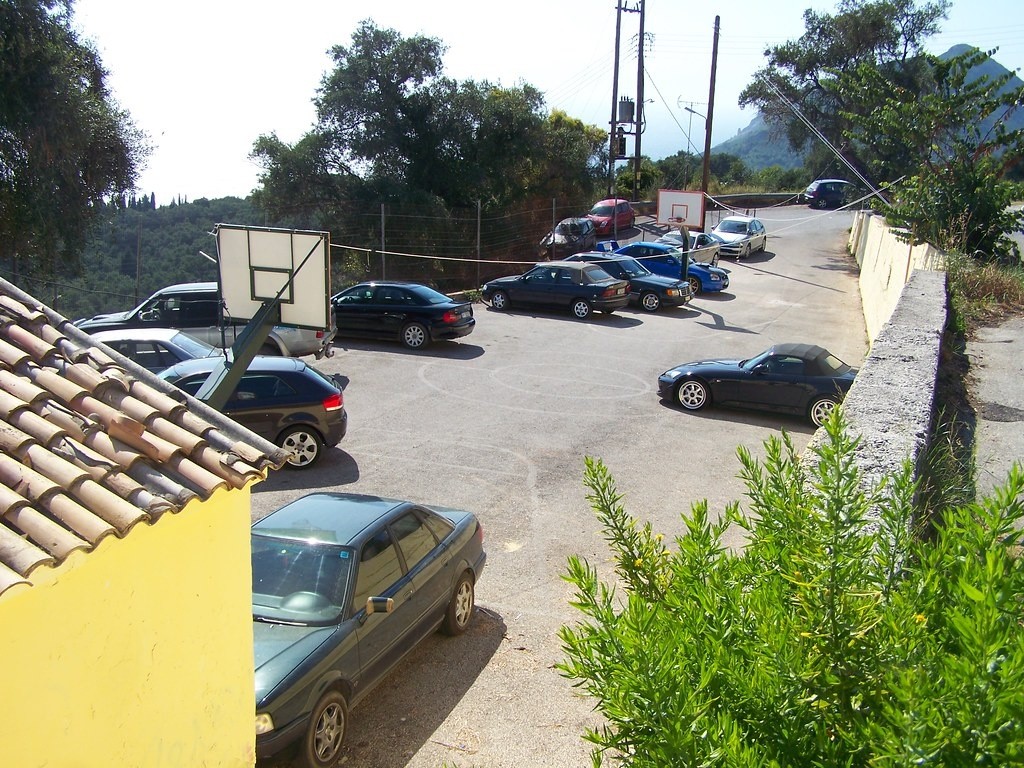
left=684, top=107, right=712, bottom=232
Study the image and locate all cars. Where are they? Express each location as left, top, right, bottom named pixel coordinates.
left=539, top=218, right=597, bottom=258
left=656, top=230, right=721, bottom=267
left=709, top=216, right=767, bottom=259
left=655, top=342, right=859, bottom=430
left=88, top=330, right=228, bottom=378
left=597, top=240, right=730, bottom=297
left=563, top=250, right=695, bottom=312
left=330, top=281, right=476, bottom=353
left=584, top=199, right=635, bottom=237
left=482, top=262, right=632, bottom=321
left=248, top=491, right=489, bottom=768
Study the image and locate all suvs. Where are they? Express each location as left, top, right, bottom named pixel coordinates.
left=72, top=281, right=338, bottom=363
left=152, top=355, right=347, bottom=472
left=805, top=179, right=867, bottom=209
